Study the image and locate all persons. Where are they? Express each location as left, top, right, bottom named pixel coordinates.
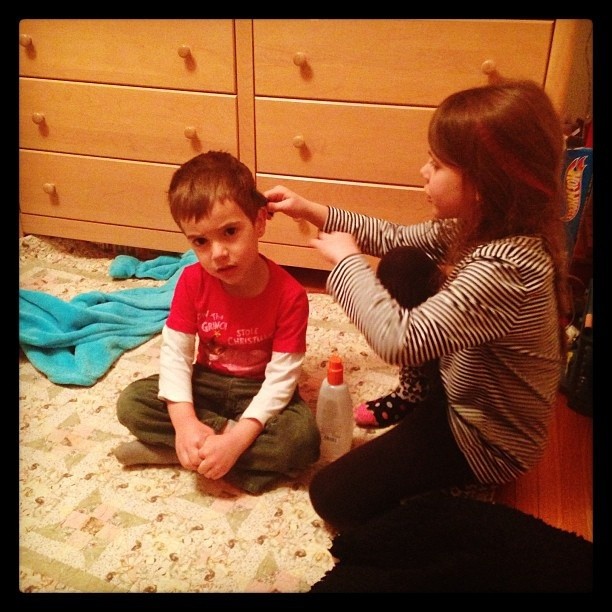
left=261, top=83, right=573, bottom=534
left=114, top=148, right=322, bottom=496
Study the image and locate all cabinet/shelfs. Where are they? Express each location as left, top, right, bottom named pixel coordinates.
left=17, top=18, right=596, bottom=276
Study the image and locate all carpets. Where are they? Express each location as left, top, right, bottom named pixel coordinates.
left=307, top=494, right=594, bottom=603
left=15, top=232, right=406, bottom=594
